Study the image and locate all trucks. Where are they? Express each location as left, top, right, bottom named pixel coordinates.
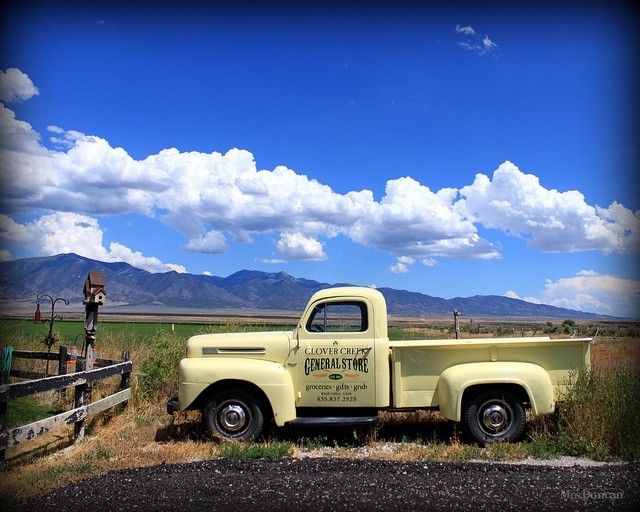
left=165, top=285, right=595, bottom=448
